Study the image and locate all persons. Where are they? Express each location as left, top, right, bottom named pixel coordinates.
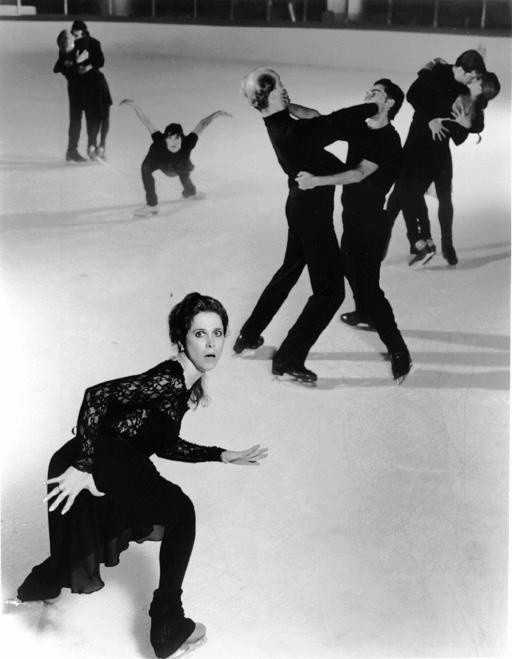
left=281, top=76, right=415, bottom=381
left=232, top=67, right=386, bottom=385
left=398, top=56, right=501, bottom=268
left=14, top=290, right=270, bottom=659
left=52, top=19, right=114, bottom=163
left=388, top=49, right=485, bottom=269
left=119, top=99, right=232, bottom=218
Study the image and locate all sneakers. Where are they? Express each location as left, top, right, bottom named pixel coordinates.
left=273, top=359, right=317, bottom=382
left=87, top=145, right=107, bottom=163
left=181, top=190, right=206, bottom=200
left=340, top=311, right=375, bottom=329
left=233, top=334, right=264, bottom=353
left=66, top=150, right=86, bottom=162
left=150, top=622, right=205, bottom=658
left=134, top=204, right=158, bottom=216
left=17, top=580, right=61, bottom=602
left=390, top=347, right=410, bottom=378
left=408, top=240, right=457, bottom=265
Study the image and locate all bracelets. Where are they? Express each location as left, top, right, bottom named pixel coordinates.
left=430, top=58, right=436, bottom=65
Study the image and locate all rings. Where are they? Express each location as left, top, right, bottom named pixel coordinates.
left=56, top=485, right=61, bottom=493
